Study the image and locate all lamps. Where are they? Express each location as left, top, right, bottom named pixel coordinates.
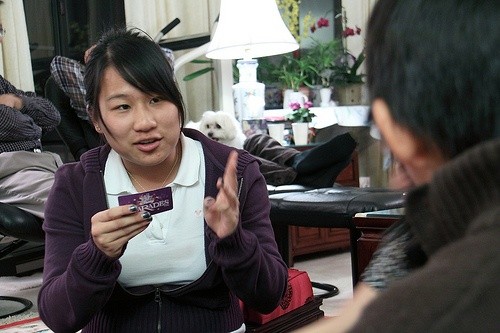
left=206, top=0, right=299, bottom=119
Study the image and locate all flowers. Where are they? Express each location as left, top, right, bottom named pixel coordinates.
left=284, top=100, right=315, bottom=123
left=276, top=0, right=316, bottom=56
left=311, top=6, right=367, bottom=82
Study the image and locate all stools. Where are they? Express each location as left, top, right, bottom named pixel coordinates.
left=268, top=177, right=407, bottom=299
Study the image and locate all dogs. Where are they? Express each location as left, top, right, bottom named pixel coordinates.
left=185, top=110, right=246, bottom=150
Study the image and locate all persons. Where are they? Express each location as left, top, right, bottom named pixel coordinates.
left=51, top=45, right=174, bottom=120
left=353, top=0, right=500, bottom=333
left=0, top=74, right=63, bottom=220
left=37, top=29, right=288, bottom=333
left=292, top=153, right=412, bottom=333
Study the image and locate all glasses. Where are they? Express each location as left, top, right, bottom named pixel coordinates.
left=0, top=27, right=7, bottom=37
left=363, top=107, right=383, bottom=141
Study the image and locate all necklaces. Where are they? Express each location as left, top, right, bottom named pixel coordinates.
left=122, top=144, right=178, bottom=191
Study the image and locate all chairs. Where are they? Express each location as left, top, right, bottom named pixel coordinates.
left=0, top=201, right=45, bottom=260
left=42, top=78, right=89, bottom=161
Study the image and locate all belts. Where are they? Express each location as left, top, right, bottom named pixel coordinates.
left=25, top=146, right=42, bottom=153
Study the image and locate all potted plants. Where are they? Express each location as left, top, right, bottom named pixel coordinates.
left=275, top=54, right=305, bottom=108
left=301, top=39, right=341, bottom=106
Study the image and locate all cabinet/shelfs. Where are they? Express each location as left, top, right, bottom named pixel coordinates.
left=265, top=104, right=373, bottom=269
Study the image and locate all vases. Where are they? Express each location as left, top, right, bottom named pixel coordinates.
left=338, top=80, right=369, bottom=105
left=290, top=121, right=308, bottom=145
